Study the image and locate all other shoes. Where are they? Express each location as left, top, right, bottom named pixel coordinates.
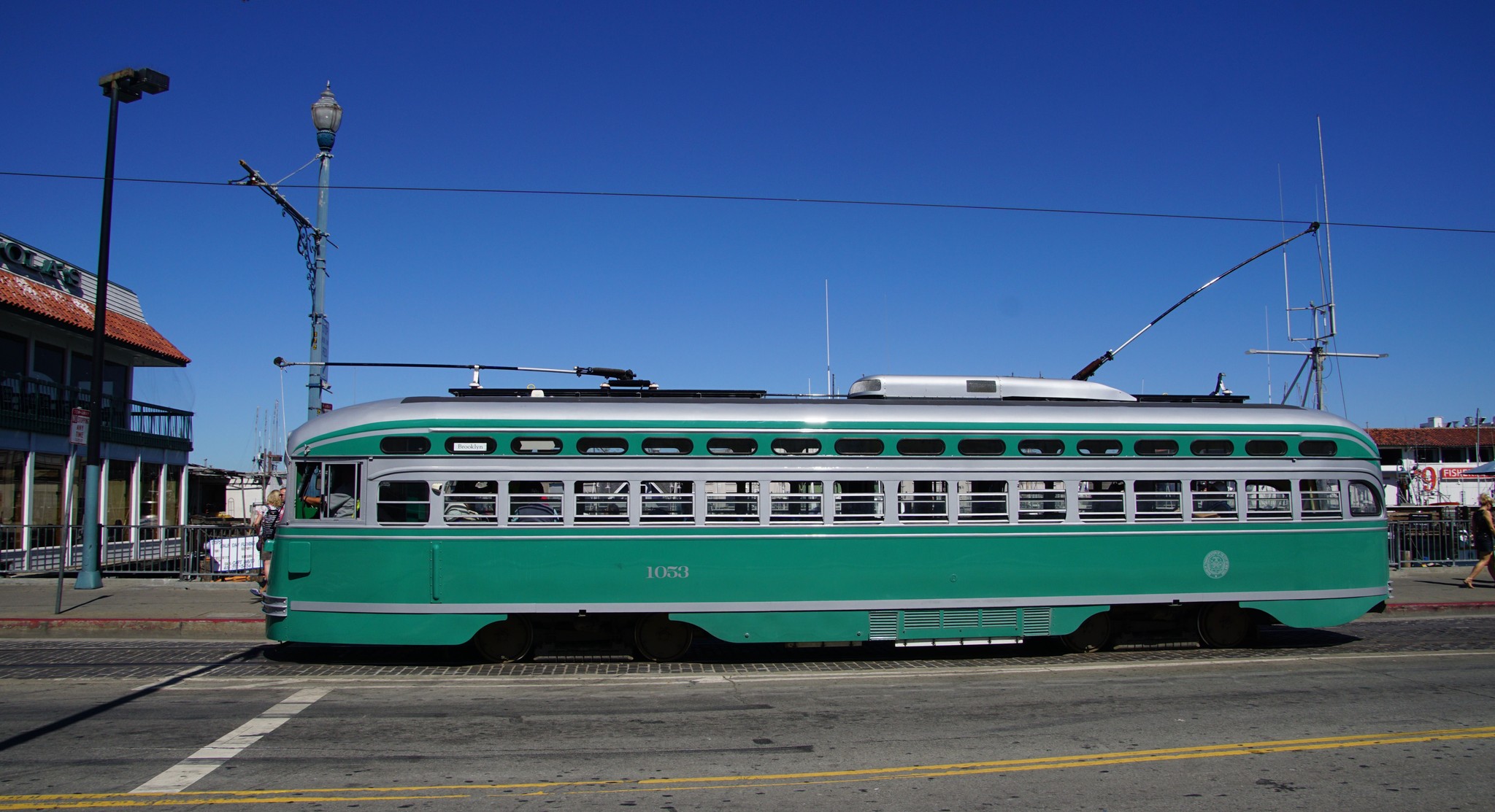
left=250, top=589, right=266, bottom=598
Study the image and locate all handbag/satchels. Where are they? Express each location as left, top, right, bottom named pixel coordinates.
left=256, top=535, right=262, bottom=552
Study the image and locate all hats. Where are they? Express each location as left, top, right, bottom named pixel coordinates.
left=1207, top=481, right=1228, bottom=496
left=1197, top=482, right=1207, bottom=487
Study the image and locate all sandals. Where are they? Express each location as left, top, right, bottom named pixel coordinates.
left=1463, top=578, right=1474, bottom=588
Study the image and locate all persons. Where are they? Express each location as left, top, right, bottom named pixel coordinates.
left=254, top=489, right=283, bottom=581
left=510, top=482, right=560, bottom=522
left=1463, top=492, right=1495, bottom=588
left=444, top=497, right=481, bottom=522
left=251, top=487, right=286, bottom=597
left=300, top=470, right=355, bottom=518
left=1205, top=482, right=1232, bottom=519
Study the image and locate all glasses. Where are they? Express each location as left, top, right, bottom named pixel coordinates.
left=1199, top=488, right=1207, bottom=490
left=1487, top=502, right=1493, bottom=507
left=279, top=494, right=286, bottom=497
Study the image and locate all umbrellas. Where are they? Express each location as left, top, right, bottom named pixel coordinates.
left=1459, top=460, right=1495, bottom=475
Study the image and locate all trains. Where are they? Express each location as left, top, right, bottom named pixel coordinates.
left=249, top=222, right=1394, bottom=650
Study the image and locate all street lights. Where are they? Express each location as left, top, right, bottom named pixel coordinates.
left=228, top=80, right=343, bottom=421
left=70, top=69, right=171, bottom=589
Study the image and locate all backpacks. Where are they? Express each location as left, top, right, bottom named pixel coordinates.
left=261, top=504, right=282, bottom=539
